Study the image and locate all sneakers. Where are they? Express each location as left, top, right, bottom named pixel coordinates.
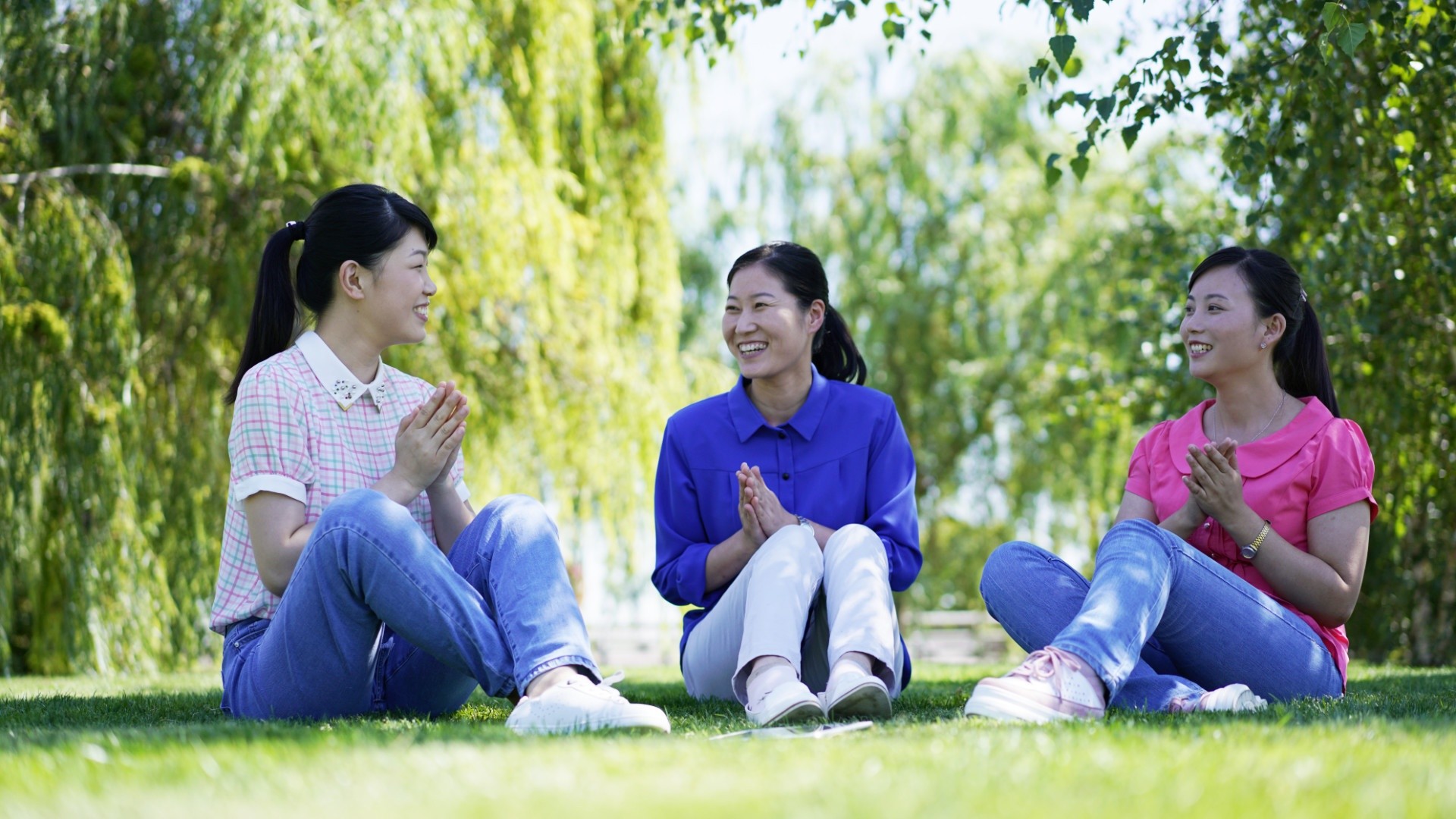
left=504, top=681, right=673, bottom=736
left=1171, top=682, right=1269, bottom=717
left=961, top=647, right=1107, bottom=726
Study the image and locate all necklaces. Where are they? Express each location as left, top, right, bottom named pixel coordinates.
left=1213, top=389, right=1286, bottom=445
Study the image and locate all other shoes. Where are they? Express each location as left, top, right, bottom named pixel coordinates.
left=825, top=671, right=894, bottom=722
left=743, top=681, right=826, bottom=727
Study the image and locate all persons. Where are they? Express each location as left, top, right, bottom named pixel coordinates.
left=209, top=183, right=672, bottom=736
left=652, top=243, right=924, bottom=727
left=966, top=246, right=1380, bottom=727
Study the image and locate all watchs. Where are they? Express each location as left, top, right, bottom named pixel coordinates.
left=1241, top=518, right=1272, bottom=560
left=794, top=513, right=816, bottom=538
